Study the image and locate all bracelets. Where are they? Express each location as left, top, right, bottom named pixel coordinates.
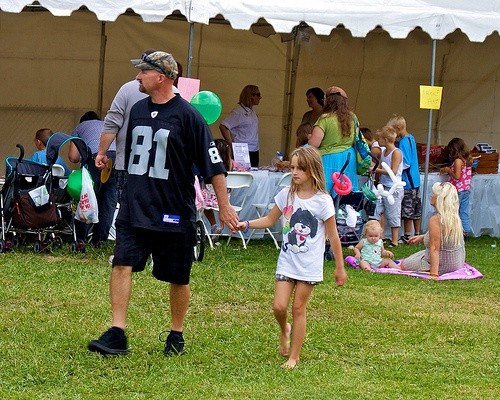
left=242, top=220, right=249, bottom=233
left=429, top=273, right=438, bottom=276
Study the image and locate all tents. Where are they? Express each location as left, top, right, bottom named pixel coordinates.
left=0, top=0, right=499, bottom=240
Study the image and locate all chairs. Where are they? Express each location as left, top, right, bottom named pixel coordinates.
left=243, top=172, right=292, bottom=251
left=36, top=164, right=77, bottom=245
left=197, top=172, right=254, bottom=249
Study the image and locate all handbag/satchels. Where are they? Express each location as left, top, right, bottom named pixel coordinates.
left=351, top=113, right=372, bottom=175
left=74, top=167, right=99, bottom=223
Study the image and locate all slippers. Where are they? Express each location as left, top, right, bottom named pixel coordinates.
left=388, top=243, right=396, bottom=247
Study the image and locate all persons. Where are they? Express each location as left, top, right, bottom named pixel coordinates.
left=307, top=87, right=373, bottom=259
left=354, top=182, right=467, bottom=280
left=301, top=88, right=326, bottom=124
left=71, top=111, right=117, bottom=253
left=94, top=48, right=159, bottom=170
left=358, top=128, right=387, bottom=244
left=221, top=84, right=261, bottom=166
left=87, top=52, right=239, bottom=356
left=441, top=138, right=472, bottom=238
left=231, top=147, right=348, bottom=368
left=30, top=126, right=70, bottom=177
left=374, top=122, right=405, bottom=247
left=296, top=122, right=313, bottom=147
left=386, top=114, right=423, bottom=244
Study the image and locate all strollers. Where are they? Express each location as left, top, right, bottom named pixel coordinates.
left=0, top=144, right=86, bottom=255
left=324, top=152, right=380, bottom=261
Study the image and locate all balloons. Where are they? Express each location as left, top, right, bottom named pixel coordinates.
left=375, top=162, right=407, bottom=206
left=332, top=171, right=351, bottom=195
left=190, top=90, right=223, bottom=125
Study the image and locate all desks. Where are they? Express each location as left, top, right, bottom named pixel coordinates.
left=356, top=173, right=500, bottom=241
left=197, top=168, right=294, bottom=242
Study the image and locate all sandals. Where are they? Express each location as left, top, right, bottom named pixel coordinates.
left=279, top=323, right=291, bottom=356
left=281, top=356, right=300, bottom=369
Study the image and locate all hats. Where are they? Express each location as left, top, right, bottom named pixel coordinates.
left=136, top=52, right=179, bottom=80
left=130, top=48, right=157, bottom=65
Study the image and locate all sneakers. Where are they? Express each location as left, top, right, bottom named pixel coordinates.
left=89, top=328, right=128, bottom=354
left=159, top=331, right=185, bottom=356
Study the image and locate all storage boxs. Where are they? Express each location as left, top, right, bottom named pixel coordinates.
left=414, top=142, right=500, bottom=174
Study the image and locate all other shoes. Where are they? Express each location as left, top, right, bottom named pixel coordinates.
left=398, top=234, right=411, bottom=244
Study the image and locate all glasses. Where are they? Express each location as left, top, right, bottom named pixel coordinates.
left=142, top=53, right=166, bottom=75
left=251, top=92, right=261, bottom=98
